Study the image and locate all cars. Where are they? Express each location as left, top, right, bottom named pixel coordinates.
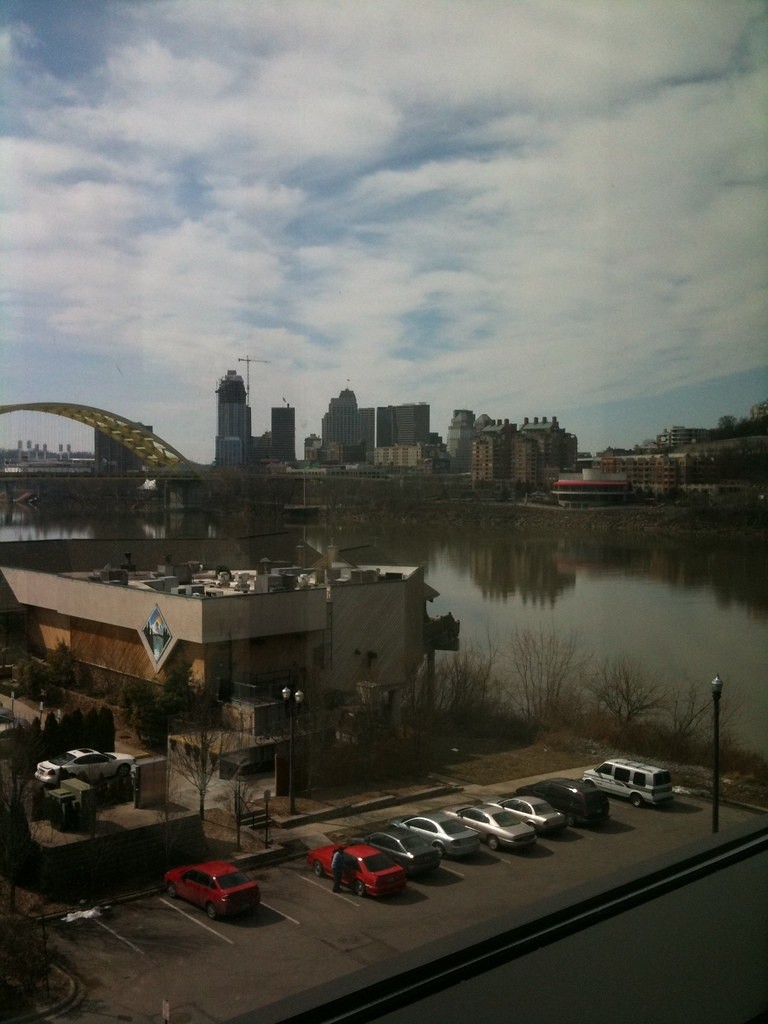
left=35, top=747, right=136, bottom=787
left=307, top=844, right=407, bottom=898
left=517, top=777, right=610, bottom=828
left=164, top=862, right=261, bottom=921
left=385, top=813, right=481, bottom=859
left=439, top=804, right=538, bottom=851
left=486, top=796, right=565, bottom=837
left=345, top=830, right=442, bottom=874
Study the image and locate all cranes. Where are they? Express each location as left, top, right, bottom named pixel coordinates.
left=238, top=355, right=272, bottom=405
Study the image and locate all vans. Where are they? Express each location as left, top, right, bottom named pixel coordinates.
left=582, top=760, right=672, bottom=808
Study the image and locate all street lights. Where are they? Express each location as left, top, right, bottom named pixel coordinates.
left=282, top=687, right=304, bottom=815
left=711, top=673, right=720, bottom=833
